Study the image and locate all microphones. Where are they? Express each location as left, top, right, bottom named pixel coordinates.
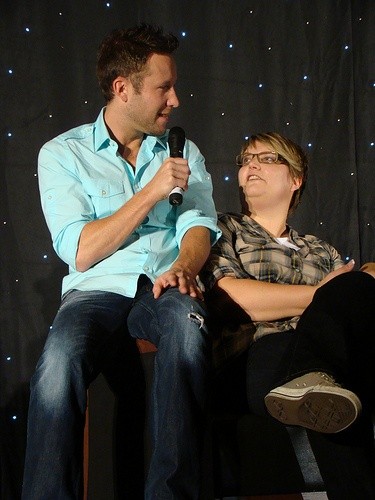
left=167, top=127, right=185, bottom=206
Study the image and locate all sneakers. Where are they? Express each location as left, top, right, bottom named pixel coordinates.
left=264, top=371, right=362, bottom=433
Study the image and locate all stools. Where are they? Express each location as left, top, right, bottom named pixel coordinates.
left=82, top=338, right=158, bottom=500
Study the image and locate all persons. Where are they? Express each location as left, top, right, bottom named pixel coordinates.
left=195, top=131, right=375, bottom=500
left=22, top=21, right=222, bottom=500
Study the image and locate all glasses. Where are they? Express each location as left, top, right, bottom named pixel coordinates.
left=236, top=150, right=284, bottom=165
